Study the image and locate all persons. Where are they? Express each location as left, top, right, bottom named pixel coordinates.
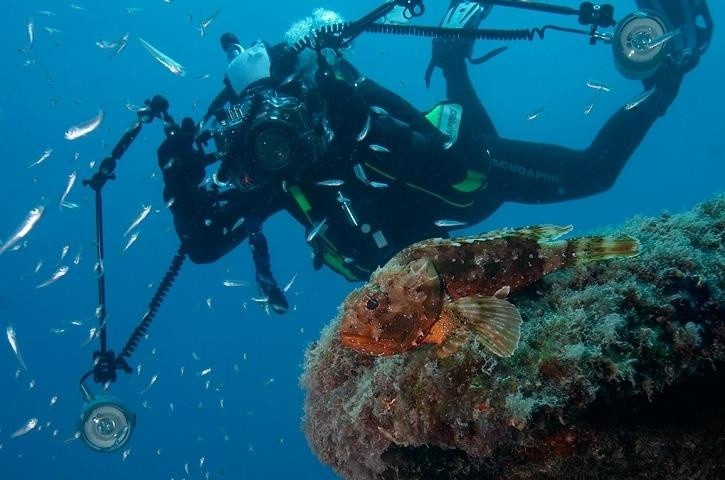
left=157, top=0, right=716, bottom=280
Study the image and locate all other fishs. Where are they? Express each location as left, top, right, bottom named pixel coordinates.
left=0, top=0, right=225, bottom=480
